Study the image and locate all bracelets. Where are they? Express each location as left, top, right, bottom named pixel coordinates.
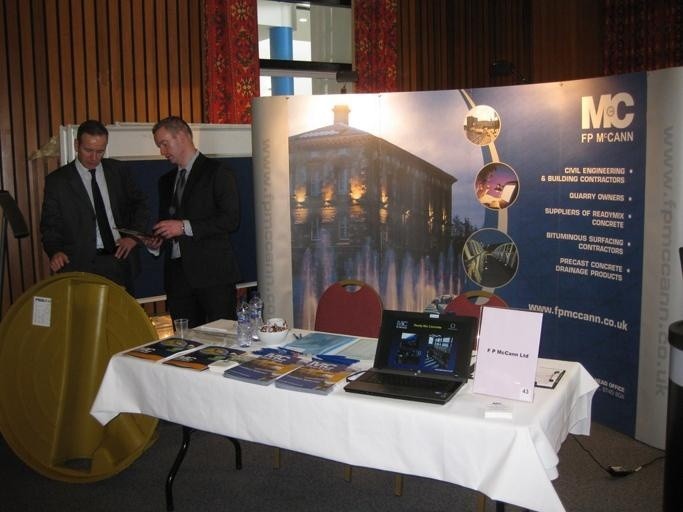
left=182, top=220, right=185, bottom=235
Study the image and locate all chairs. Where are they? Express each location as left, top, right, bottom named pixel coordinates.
left=389, top=286, right=511, bottom=512
left=264, top=277, right=386, bottom=487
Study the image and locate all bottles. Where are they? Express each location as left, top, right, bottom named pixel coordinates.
left=249, top=291, right=263, bottom=341
left=235, top=297, right=251, bottom=347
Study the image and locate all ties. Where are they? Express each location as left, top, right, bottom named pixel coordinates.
left=169, top=169, right=186, bottom=244
left=87, top=169, right=117, bottom=256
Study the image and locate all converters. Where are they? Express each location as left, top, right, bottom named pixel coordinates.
left=610, top=466, right=630, bottom=478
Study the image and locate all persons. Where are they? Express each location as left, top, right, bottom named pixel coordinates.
left=39, top=119, right=150, bottom=298
left=137, top=117, right=240, bottom=333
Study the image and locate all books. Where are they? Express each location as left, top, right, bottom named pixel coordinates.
left=275, top=359, right=362, bottom=395
left=224, top=347, right=313, bottom=386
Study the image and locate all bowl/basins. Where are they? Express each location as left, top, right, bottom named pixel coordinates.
left=257, top=325, right=289, bottom=345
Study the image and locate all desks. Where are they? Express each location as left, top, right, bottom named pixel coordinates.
left=85, top=314, right=602, bottom=511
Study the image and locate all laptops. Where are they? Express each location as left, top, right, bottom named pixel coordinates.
left=345, top=311, right=477, bottom=404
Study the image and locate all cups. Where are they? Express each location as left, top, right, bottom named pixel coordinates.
left=173, top=319, right=188, bottom=338
